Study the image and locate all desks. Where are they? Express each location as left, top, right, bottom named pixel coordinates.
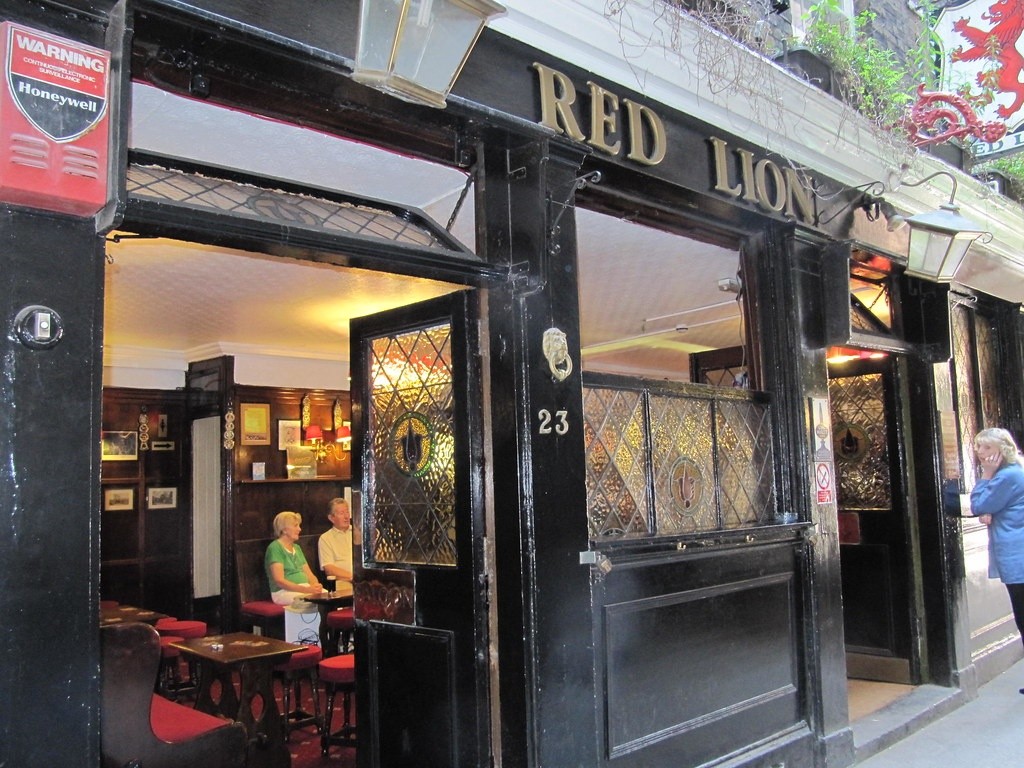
left=301, top=589, right=353, bottom=659
left=169, top=631, right=292, bottom=768
left=100, top=604, right=168, bottom=626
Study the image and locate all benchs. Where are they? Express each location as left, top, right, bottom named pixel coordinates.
left=235, top=534, right=334, bottom=634
left=101, top=622, right=249, bottom=768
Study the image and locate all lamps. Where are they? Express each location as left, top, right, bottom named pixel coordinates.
left=353, top=1, right=510, bottom=110
left=810, top=181, right=906, bottom=232
left=889, top=163, right=993, bottom=285
left=306, top=426, right=352, bottom=463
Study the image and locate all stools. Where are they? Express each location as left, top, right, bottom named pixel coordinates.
left=274, top=645, right=325, bottom=743
left=101, top=601, right=119, bottom=608
left=327, top=609, right=354, bottom=652
left=154, top=617, right=207, bottom=703
left=318, top=653, right=356, bottom=768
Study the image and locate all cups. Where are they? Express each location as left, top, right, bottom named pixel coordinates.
left=327, top=576, right=336, bottom=595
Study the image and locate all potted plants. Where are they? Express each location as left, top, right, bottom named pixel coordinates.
left=769, top=0, right=975, bottom=174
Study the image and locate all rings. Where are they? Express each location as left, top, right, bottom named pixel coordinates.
left=988, top=460, right=992, bottom=462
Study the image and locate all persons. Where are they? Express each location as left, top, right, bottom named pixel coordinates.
left=971, top=428, right=1024, bottom=694
left=265, top=512, right=329, bottom=607
left=318, top=498, right=352, bottom=592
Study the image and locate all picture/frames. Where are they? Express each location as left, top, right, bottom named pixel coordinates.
left=145, top=485, right=179, bottom=511
left=102, top=430, right=139, bottom=461
left=342, top=420, right=352, bottom=452
left=103, top=487, right=135, bottom=511
left=241, top=404, right=271, bottom=446
left=277, top=419, right=302, bottom=450
